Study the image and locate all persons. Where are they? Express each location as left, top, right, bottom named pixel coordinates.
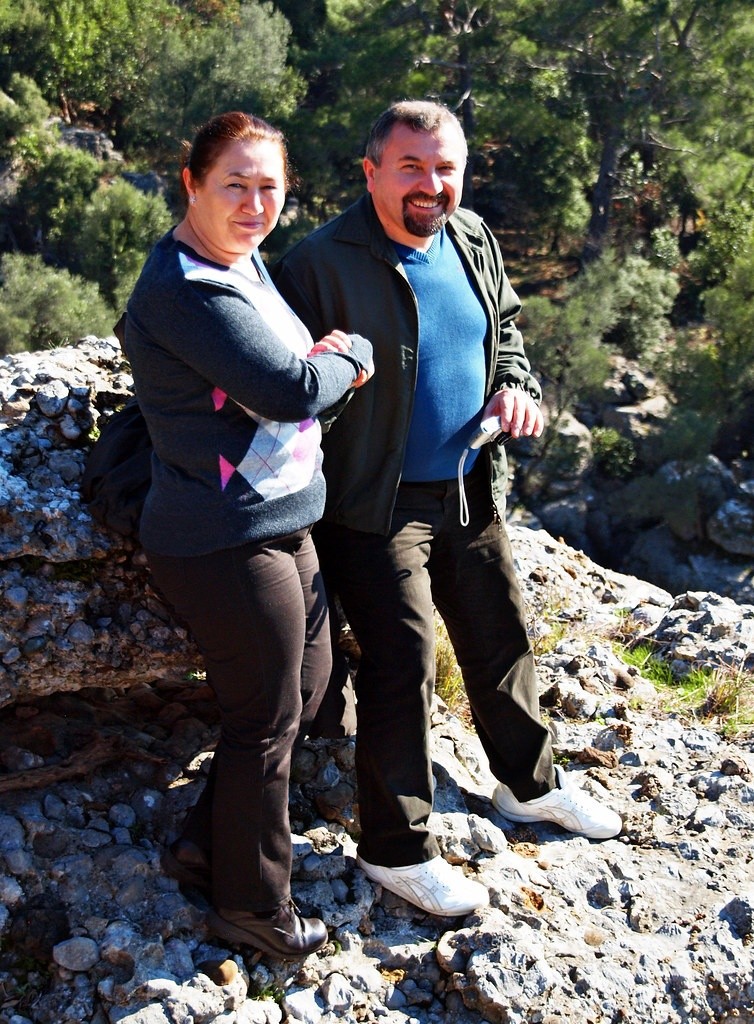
left=111, top=110, right=373, bottom=957
left=274, top=100, right=621, bottom=914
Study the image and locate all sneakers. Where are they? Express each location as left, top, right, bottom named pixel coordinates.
left=357, top=853, right=489, bottom=916
left=492, top=765, right=622, bottom=838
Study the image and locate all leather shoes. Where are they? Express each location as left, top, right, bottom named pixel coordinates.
left=203, top=898, right=328, bottom=959
left=160, top=831, right=213, bottom=890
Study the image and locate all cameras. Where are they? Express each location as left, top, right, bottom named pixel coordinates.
left=468, top=415, right=515, bottom=450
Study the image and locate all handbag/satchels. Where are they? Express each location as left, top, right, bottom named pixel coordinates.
left=81, top=398, right=153, bottom=543
